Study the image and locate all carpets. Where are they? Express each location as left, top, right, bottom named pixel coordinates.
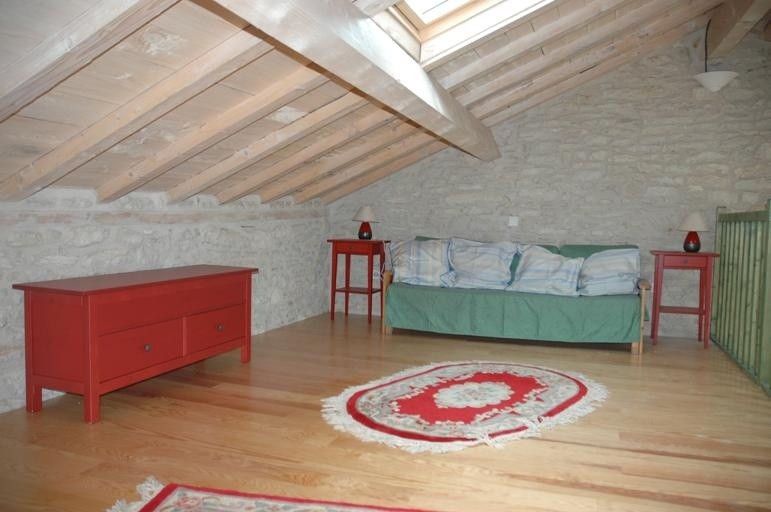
left=104, top=474, right=435, bottom=512
left=320, top=360, right=610, bottom=456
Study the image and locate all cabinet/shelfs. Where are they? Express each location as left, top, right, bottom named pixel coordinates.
left=10, top=264, right=258, bottom=425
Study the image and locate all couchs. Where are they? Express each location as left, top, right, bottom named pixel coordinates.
left=382, top=235, right=651, bottom=361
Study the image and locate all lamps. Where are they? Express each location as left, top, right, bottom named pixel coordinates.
left=351, top=206, right=380, bottom=239
left=676, top=213, right=709, bottom=253
left=691, top=17, right=739, bottom=93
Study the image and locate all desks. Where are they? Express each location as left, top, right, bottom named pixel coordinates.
left=326, top=239, right=390, bottom=323
left=649, top=250, right=720, bottom=348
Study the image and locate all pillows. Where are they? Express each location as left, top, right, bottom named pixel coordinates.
left=386, top=236, right=642, bottom=298
